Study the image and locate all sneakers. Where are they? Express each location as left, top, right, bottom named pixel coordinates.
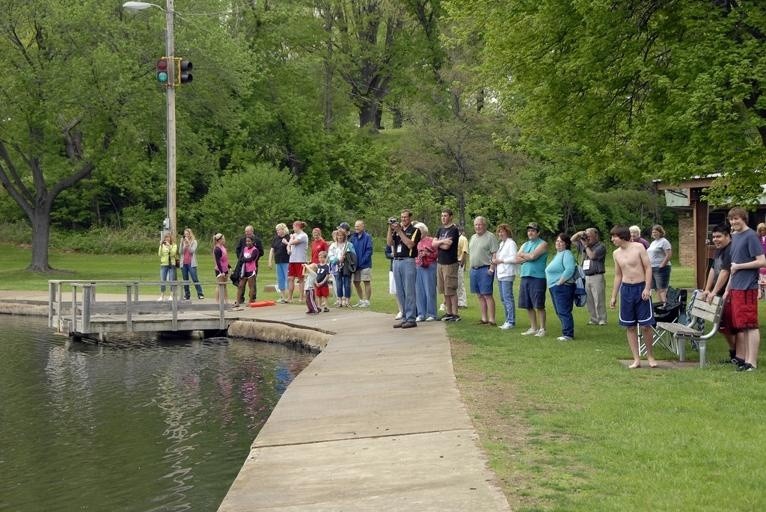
left=497, top=321, right=515, bottom=330
left=393, top=303, right=468, bottom=322
left=276, top=296, right=371, bottom=315
left=557, top=336, right=574, bottom=342
left=520, top=328, right=546, bottom=338
left=724, top=350, right=758, bottom=372
left=158, top=294, right=205, bottom=303
left=587, top=320, right=608, bottom=326
left=393, top=321, right=417, bottom=329
left=477, top=320, right=497, bottom=327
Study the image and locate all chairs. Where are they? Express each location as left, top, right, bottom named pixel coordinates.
left=635, top=285, right=683, bottom=359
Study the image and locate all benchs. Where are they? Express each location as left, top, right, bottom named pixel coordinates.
left=657, top=289, right=725, bottom=369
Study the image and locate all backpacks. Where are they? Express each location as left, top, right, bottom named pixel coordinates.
left=562, top=251, right=588, bottom=307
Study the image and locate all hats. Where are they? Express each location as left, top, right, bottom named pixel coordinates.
left=526, top=222, right=540, bottom=231
left=336, top=222, right=350, bottom=231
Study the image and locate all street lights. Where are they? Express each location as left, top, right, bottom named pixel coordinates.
left=120, top=1, right=178, bottom=241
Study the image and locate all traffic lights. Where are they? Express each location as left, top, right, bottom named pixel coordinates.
left=157, top=58, right=169, bottom=85
left=180, top=58, right=193, bottom=83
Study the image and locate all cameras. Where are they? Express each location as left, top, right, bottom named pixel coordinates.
left=390, top=218, right=398, bottom=224
left=581, top=234, right=587, bottom=240
